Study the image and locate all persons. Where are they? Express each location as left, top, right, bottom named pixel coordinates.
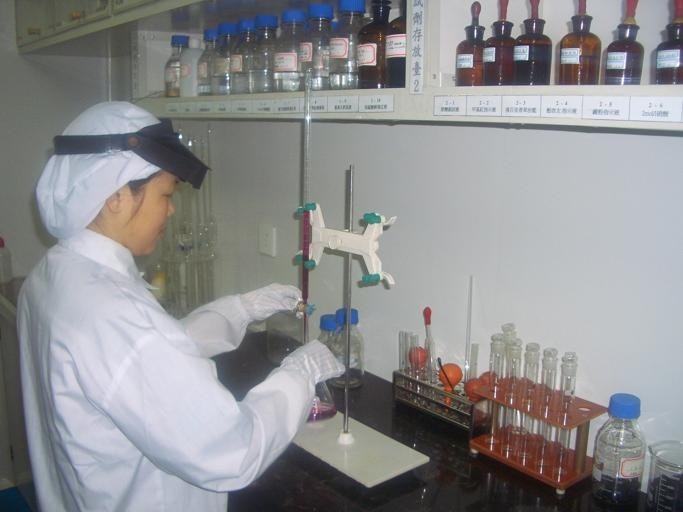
left=17, top=101, right=346, bottom=511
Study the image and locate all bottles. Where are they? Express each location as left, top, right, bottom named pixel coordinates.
left=395, top=306, right=479, bottom=421
left=453, top=0, right=683, bottom=86
left=588, top=393, right=647, bottom=511
left=486, top=323, right=578, bottom=478
left=162, top=0, right=407, bottom=99
left=317, top=304, right=366, bottom=392
left=302, top=381, right=338, bottom=425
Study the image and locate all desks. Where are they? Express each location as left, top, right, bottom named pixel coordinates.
left=213, top=331, right=651, bottom=510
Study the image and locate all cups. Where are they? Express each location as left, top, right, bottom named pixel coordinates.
left=262, top=313, right=301, bottom=367
left=643, top=440, right=683, bottom=510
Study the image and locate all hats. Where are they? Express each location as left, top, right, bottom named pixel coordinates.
left=34, top=101, right=163, bottom=239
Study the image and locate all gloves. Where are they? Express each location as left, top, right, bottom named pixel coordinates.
left=279, top=338, right=346, bottom=384
left=239, top=283, right=304, bottom=320
